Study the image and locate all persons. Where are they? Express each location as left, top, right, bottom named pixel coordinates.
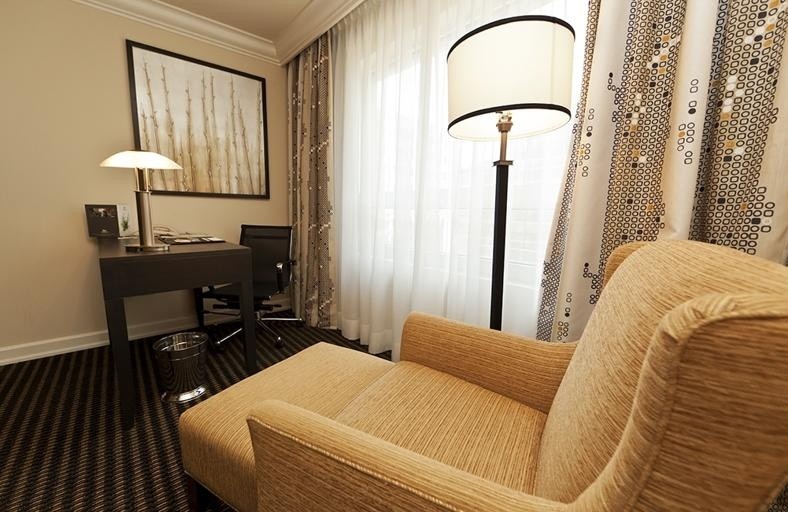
left=89, top=208, right=113, bottom=233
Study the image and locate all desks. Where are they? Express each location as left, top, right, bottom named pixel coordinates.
left=99, top=235, right=257, bottom=432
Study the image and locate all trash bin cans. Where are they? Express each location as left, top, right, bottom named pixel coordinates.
left=153, top=332, right=209, bottom=404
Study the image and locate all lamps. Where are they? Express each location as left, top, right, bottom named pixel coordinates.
left=444, top=15, right=576, bottom=333
left=100, top=149, right=183, bottom=254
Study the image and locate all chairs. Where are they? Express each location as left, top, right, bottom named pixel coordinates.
left=177, top=234, right=788, bottom=512
left=202, top=224, right=305, bottom=350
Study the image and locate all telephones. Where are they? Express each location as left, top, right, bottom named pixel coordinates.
left=153, top=224, right=178, bottom=237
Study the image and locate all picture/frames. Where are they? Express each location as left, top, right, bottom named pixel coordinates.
left=127, top=39, right=270, bottom=200
